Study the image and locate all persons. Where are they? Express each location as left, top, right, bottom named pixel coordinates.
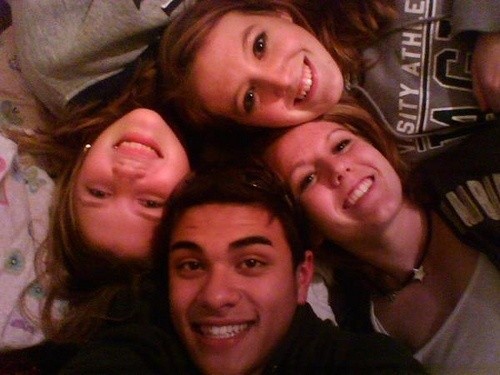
left=140, top=0, right=500, bottom=270
left=245, top=102, right=500, bottom=374
left=9, top=0, right=228, bottom=338
left=79, top=158, right=426, bottom=373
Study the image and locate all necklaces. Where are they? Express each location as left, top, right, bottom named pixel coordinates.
left=366, top=199, right=433, bottom=294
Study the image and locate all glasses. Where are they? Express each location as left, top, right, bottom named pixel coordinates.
left=187, top=168, right=309, bottom=247
